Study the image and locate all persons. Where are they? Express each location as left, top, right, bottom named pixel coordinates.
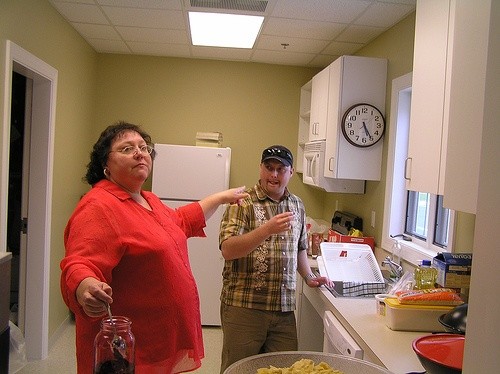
left=61, top=121, right=249, bottom=374
left=219, top=145, right=335, bottom=374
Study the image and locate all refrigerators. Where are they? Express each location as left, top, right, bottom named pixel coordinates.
left=152, top=144, right=232, bottom=325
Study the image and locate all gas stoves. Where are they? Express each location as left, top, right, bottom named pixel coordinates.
left=307, top=209, right=363, bottom=261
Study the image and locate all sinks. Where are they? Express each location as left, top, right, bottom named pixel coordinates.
left=326, top=281, right=392, bottom=297
left=312, top=268, right=386, bottom=283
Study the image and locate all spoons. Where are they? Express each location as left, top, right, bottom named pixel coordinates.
left=107, top=302, right=129, bottom=369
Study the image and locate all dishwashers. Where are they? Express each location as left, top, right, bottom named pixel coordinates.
left=322, top=311, right=363, bottom=360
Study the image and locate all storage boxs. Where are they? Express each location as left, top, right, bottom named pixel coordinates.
left=433, top=252, right=472, bottom=288
left=328, top=228, right=375, bottom=253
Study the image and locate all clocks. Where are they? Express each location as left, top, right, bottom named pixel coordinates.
left=341, top=103, right=386, bottom=148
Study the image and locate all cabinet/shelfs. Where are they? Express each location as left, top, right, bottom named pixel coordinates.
left=294, top=271, right=324, bottom=353
left=296, top=55, right=388, bottom=181
left=404, top=0, right=491, bottom=214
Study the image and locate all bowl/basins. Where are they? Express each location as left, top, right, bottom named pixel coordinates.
left=221, top=350, right=391, bottom=374
left=438, top=304, right=469, bottom=334
left=375, top=294, right=393, bottom=317
left=413, top=334, right=467, bottom=374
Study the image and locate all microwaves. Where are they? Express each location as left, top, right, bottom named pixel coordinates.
left=302, top=141, right=365, bottom=195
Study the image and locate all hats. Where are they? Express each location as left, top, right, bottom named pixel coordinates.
left=262, top=145, right=293, bottom=167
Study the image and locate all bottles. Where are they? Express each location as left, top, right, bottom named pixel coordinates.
left=414, top=260, right=438, bottom=290
left=93, top=316, right=136, bottom=374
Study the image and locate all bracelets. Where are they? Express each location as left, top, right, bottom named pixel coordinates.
left=305, top=274, right=316, bottom=282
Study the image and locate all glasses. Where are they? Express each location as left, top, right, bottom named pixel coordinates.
left=110, top=145, right=154, bottom=155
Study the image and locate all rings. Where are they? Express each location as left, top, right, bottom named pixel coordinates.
left=285, top=222, right=287, bottom=225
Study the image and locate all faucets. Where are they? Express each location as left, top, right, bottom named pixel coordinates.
left=381, top=255, right=402, bottom=279
